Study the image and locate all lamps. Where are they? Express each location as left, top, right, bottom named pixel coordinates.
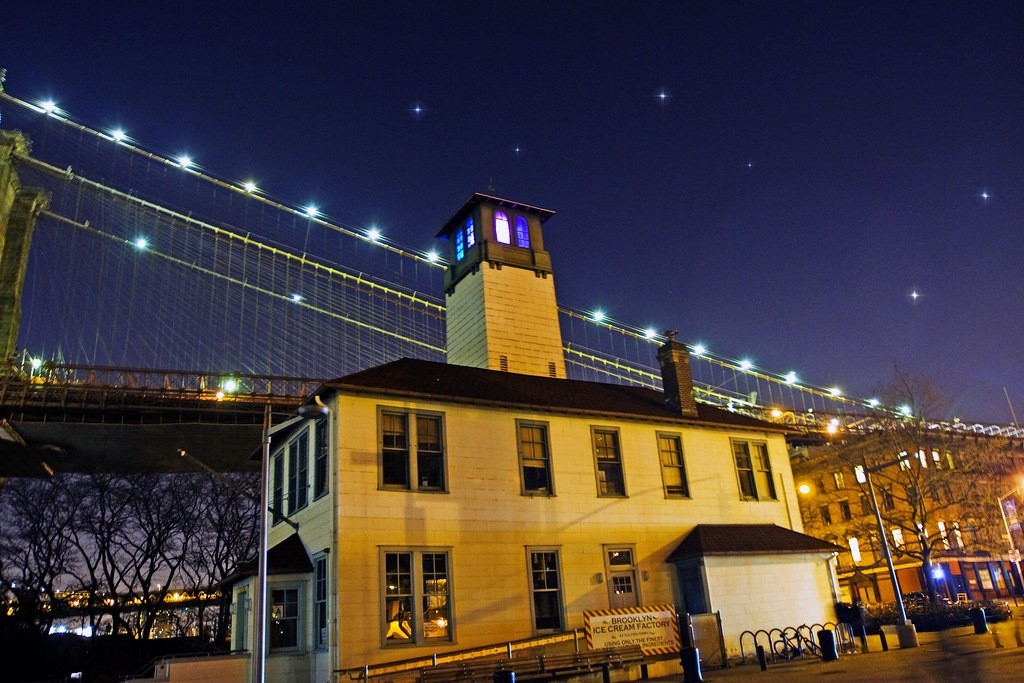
left=597, top=573, right=603, bottom=583
left=644, top=571, right=649, bottom=582
left=299, top=404, right=329, bottom=420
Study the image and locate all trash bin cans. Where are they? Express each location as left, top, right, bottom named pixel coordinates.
left=835, top=602, right=865, bottom=636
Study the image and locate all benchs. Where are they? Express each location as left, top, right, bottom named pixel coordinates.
left=419, top=643, right=656, bottom=683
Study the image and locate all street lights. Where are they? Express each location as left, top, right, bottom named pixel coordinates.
left=828, top=418, right=921, bottom=649
left=222, top=371, right=275, bottom=681
